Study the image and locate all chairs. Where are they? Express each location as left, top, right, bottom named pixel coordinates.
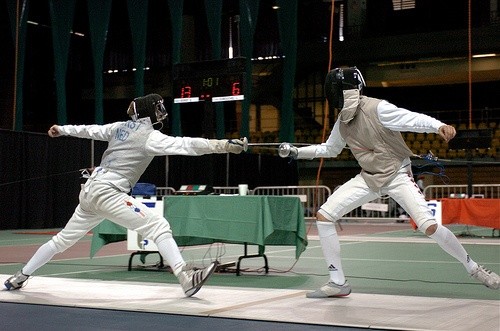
left=225, top=122, right=500, bottom=198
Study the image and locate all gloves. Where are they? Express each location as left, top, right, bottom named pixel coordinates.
left=288, top=146, right=297, bottom=159
left=227, top=139, right=244, bottom=154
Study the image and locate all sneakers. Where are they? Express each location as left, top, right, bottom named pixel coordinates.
left=4, top=268, right=30, bottom=291
left=306, top=280, right=352, bottom=299
left=177, top=262, right=218, bottom=298
left=468, top=263, right=500, bottom=290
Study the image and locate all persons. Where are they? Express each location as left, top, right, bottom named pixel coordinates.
left=288, top=66, right=500, bottom=298
left=4, top=94, right=243, bottom=297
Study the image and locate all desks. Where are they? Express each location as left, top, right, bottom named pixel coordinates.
left=409, top=198, right=500, bottom=237
left=90, top=194, right=308, bottom=277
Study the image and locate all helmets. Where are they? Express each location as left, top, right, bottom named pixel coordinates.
left=127, top=94, right=168, bottom=131
left=324, top=66, right=367, bottom=111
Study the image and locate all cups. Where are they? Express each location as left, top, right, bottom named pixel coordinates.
left=239, top=184, right=248, bottom=196
